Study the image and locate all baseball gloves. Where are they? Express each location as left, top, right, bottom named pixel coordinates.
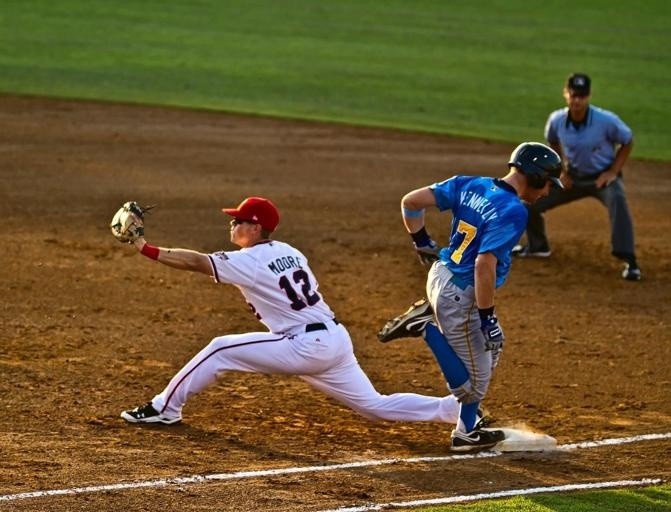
left=111, top=200, right=145, bottom=242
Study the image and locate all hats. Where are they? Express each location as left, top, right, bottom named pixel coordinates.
left=222, top=196, right=280, bottom=232
left=567, top=72, right=592, bottom=97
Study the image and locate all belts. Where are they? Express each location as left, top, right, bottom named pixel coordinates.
left=287, top=317, right=341, bottom=338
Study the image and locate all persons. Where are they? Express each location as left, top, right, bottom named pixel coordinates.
left=375, top=139, right=567, bottom=454
left=111, top=195, right=494, bottom=427
left=511, top=71, right=645, bottom=281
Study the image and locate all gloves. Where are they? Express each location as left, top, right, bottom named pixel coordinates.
left=414, top=239, right=441, bottom=265
left=480, top=316, right=505, bottom=355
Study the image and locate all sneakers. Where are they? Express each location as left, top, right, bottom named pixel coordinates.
left=621, top=262, right=642, bottom=281
left=511, top=243, right=552, bottom=258
left=474, top=401, right=497, bottom=426
left=377, top=297, right=435, bottom=344
left=449, top=427, right=505, bottom=455
left=120, top=401, right=182, bottom=425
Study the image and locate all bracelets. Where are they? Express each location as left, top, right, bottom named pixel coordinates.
left=140, top=242, right=160, bottom=262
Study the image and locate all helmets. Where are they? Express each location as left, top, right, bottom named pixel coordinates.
left=507, top=141, right=566, bottom=190
